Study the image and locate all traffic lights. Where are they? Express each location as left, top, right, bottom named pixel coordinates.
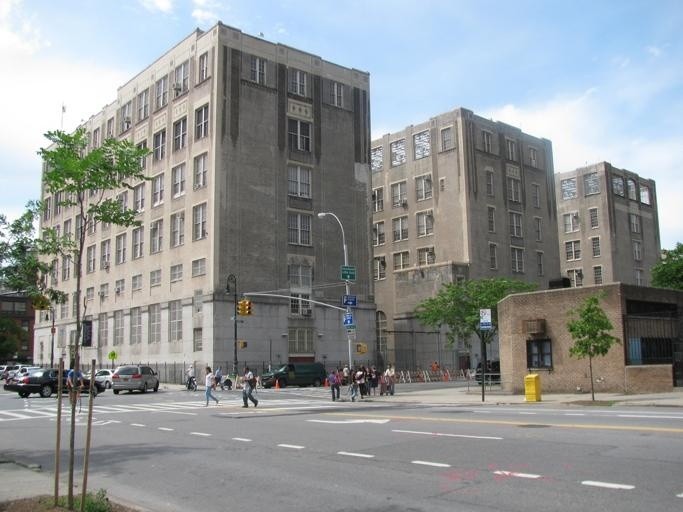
left=244, top=300, right=251, bottom=315
left=236, top=299, right=243, bottom=317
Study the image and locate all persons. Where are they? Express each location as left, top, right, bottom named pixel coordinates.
left=431, top=361, right=440, bottom=375
left=203, top=367, right=218, bottom=407
left=241, top=367, right=257, bottom=408
left=215, top=366, right=224, bottom=390
left=64, top=366, right=84, bottom=399
left=184, top=365, right=195, bottom=391
left=327, top=364, right=396, bottom=402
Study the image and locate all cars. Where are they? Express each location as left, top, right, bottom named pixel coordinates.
left=217, top=373, right=250, bottom=391
left=0, top=363, right=117, bottom=397
left=110, top=364, right=159, bottom=395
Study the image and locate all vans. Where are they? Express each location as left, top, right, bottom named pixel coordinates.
left=259, top=362, right=325, bottom=388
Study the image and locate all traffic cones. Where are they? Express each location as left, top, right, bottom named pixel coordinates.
left=323, top=378, right=328, bottom=389
left=274, top=380, right=280, bottom=391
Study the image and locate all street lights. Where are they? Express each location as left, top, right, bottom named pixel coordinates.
left=316, top=211, right=353, bottom=370
left=224, top=273, right=238, bottom=377
left=43, top=305, right=56, bottom=369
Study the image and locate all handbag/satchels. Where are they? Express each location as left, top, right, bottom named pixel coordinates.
left=248, top=377, right=257, bottom=388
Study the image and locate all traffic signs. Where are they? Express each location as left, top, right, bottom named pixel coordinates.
left=341, top=264, right=356, bottom=280
left=342, top=294, right=357, bottom=306
left=343, top=313, right=352, bottom=324
left=345, top=325, right=357, bottom=336
left=479, top=307, right=491, bottom=330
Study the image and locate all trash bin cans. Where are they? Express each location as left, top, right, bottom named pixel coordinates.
left=523, top=373, right=541, bottom=402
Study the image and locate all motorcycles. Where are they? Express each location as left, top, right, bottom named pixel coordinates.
left=183, top=375, right=197, bottom=392
